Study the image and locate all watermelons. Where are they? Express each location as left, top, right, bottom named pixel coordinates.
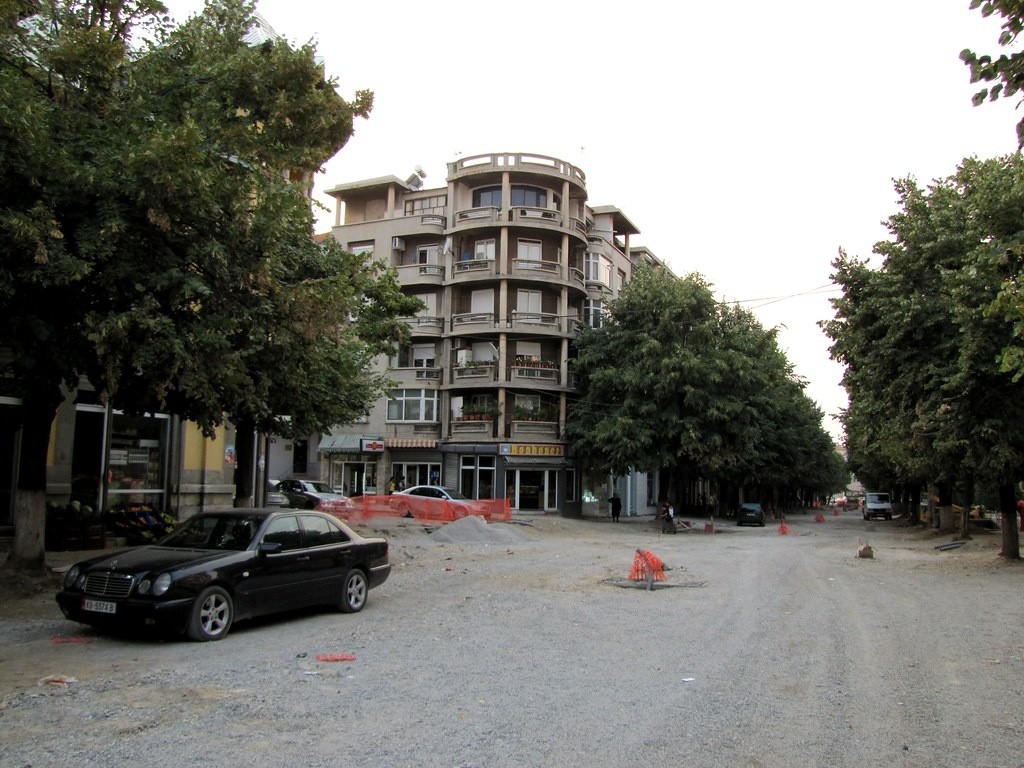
left=45, top=500, right=94, bottom=514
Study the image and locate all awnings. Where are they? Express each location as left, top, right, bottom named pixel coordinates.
left=317, top=435, right=378, bottom=453
left=384, top=439, right=437, bottom=448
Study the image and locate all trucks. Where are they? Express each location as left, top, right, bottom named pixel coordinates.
left=861, top=492, right=893, bottom=521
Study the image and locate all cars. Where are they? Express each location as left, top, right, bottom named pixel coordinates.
left=273, top=478, right=356, bottom=519
left=233, top=467, right=290, bottom=510
left=389, top=484, right=492, bottom=522
left=735, top=503, right=766, bottom=527
left=53, top=508, right=392, bottom=643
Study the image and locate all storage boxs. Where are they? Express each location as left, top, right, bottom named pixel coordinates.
left=109, top=500, right=174, bottom=544
left=45, top=523, right=106, bottom=551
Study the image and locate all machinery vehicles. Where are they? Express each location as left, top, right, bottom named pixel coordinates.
left=919, top=491, right=985, bottom=531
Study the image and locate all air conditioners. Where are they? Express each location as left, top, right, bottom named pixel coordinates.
left=455, top=337, right=466, bottom=350
left=392, top=237, right=405, bottom=251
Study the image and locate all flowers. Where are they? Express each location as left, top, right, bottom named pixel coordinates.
left=512, top=356, right=554, bottom=366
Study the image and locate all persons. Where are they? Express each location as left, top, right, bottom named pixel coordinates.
left=398, top=477, right=406, bottom=492
left=697, top=492, right=718, bottom=516
left=660, top=501, right=677, bottom=534
left=1017, top=497, right=1024, bottom=531
left=388, top=477, right=395, bottom=495
left=608, top=493, right=621, bottom=522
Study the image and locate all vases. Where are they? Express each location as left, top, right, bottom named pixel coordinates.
left=456, top=417, right=463, bottom=421
left=513, top=362, right=554, bottom=368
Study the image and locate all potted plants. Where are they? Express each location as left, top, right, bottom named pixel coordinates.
left=494, top=311, right=499, bottom=327
left=505, top=405, right=554, bottom=424
left=465, top=361, right=495, bottom=366
left=463, top=404, right=501, bottom=420
left=507, top=314, right=512, bottom=328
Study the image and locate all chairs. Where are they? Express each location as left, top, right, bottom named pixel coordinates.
left=226, top=525, right=250, bottom=550
